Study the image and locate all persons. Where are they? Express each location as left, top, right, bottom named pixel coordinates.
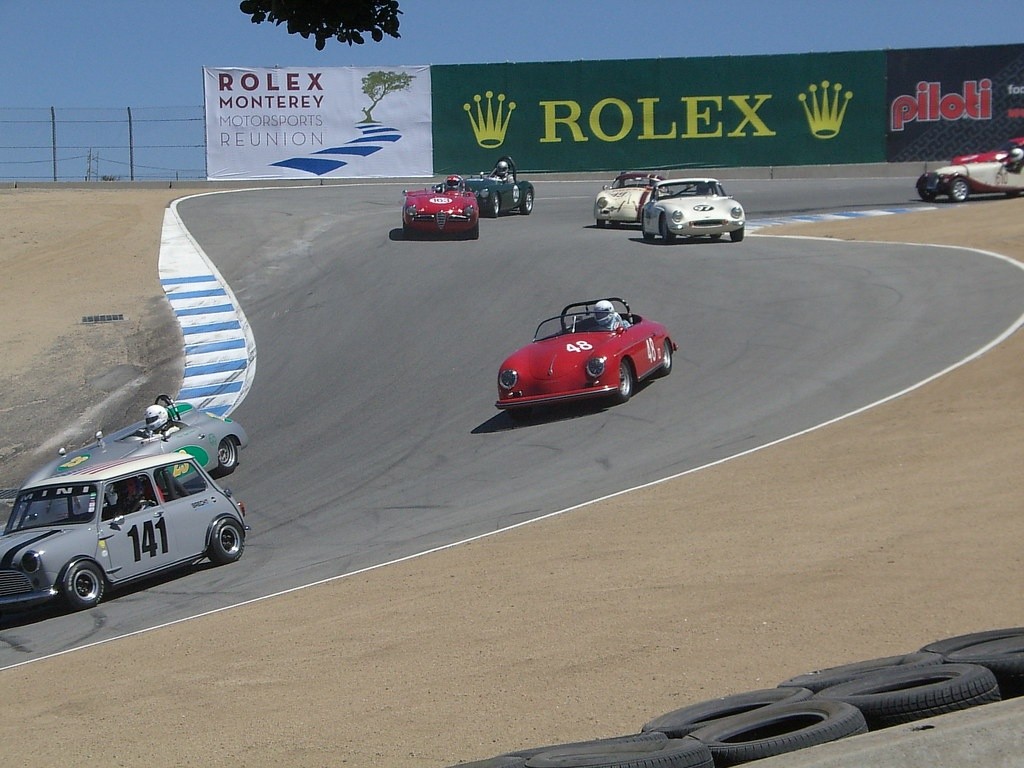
left=586, top=300, right=631, bottom=330
left=695, top=183, right=711, bottom=195
left=1005, top=148, right=1024, bottom=174
left=145, top=405, right=180, bottom=440
left=110, top=476, right=156, bottom=518
left=445, top=174, right=461, bottom=191
left=494, top=160, right=509, bottom=178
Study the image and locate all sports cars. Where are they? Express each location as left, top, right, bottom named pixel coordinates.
left=495, top=297, right=678, bottom=422
left=915, top=138, right=1024, bottom=204
left=640, top=176, right=746, bottom=244
left=0, top=452, right=251, bottom=615
left=17, top=393, right=249, bottom=523
left=402, top=174, right=480, bottom=240
left=432, top=156, right=535, bottom=219
left=594, top=171, right=672, bottom=228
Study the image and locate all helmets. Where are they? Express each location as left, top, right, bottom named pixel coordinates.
left=496, top=161, right=509, bottom=175
left=146, top=405, right=168, bottom=431
left=446, top=175, right=463, bottom=189
left=594, top=299, right=615, bottom=326
left=696, top=182, right=710, bottom=196
left=1009, top=148, right=1024, bottom=162
